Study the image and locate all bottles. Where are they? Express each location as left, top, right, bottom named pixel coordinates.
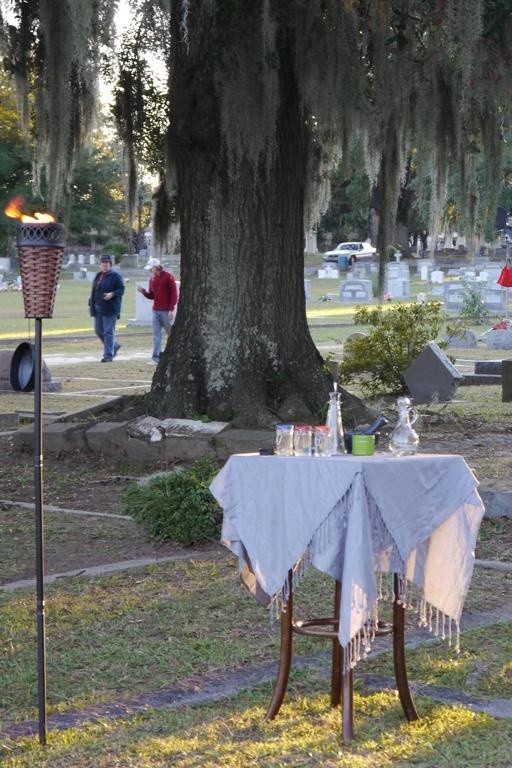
left=386, top=398, right=420, bottom=457
left=325, top=391, right=348, bottom=456
left=275, top=423, right=334, bottom=459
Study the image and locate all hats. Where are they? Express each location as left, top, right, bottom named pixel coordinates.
left=100, top=255, right=112, bottom=262
left=144, top=258, right=160, bottom=269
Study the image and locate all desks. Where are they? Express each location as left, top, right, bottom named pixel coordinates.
left=228, top=444, right=468, bottom=754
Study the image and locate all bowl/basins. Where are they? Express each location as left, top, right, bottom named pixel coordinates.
left=341, top=428, right=381, bottom=453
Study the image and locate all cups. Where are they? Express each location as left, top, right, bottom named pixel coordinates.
left=351, top=433, right=377, bottom=457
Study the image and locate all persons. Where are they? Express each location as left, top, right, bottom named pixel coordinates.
left=88, top=253, right=126, bottom=364
left=135, top=257, right=178, bottom=365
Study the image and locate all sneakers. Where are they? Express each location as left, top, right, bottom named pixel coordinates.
left=147, top=359, right=159, bottom=365
left=102, top=358, right=112, bottom=362
left=113, top=344, right=120, bottom=356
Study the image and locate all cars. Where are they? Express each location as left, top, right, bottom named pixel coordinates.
left=320, top=240, right=377, bottom=266
